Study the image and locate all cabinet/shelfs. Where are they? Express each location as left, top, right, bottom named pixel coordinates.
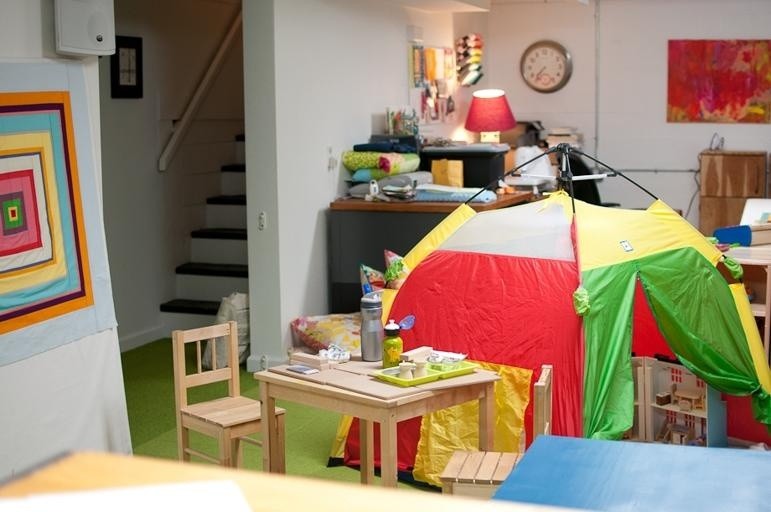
left=719, top=247, right=771, bottom=363
left=698, top=150, right=767, bottom=235
left=328, top=192, right=535, bottom=315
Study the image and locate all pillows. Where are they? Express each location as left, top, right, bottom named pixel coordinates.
left=359, top=263, right=386, bottom=296
left=383, top=249, right=409, bottom=289
left=290, top=311, right=363, bottom=359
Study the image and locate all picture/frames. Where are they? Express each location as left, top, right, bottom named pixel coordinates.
left=110, top=36, right=144, bottom=100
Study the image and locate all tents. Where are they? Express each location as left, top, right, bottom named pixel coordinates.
left=323, top=142, right=770, bottom=490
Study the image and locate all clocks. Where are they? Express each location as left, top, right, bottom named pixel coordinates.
left=519, top=41, right=572, bottom=93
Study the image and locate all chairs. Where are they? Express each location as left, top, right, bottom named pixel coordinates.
left=439, top=365, right=554, bottom=494
left=171, top=322, right=286, bottom=474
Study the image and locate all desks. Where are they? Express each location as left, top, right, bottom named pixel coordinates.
left=493, top=435, right=771, bottom=511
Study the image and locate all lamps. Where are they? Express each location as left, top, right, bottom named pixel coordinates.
left=465, top=88, right=516, bottom=144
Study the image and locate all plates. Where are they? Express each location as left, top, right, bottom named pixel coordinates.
left=421, top=359, right=478, bottom=379
left=369, top=365, right=439, bottom=386
